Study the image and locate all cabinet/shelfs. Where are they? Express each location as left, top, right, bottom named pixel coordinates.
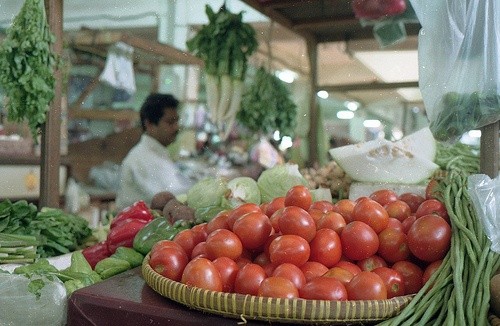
left=59, top=31, right=205, bottom=201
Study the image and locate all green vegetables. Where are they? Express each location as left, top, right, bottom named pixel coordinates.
left=0, top=197, right=91, bottom=264
left=234, top=65, right=298, bottom=144
left=0, top=0, right=64, bottom=146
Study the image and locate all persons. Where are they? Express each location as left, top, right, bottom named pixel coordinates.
left=115, top=92, right=264, bottom=215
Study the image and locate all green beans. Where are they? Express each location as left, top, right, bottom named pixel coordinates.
left=378, top=147, right=500, bottom=326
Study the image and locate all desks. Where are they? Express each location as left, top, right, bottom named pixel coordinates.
left=69, top=264, right=289, bottom=326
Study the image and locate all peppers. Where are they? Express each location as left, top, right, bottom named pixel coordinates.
left=430, top=90, right=500, bottom=140
left=14, top=199, right=190, bottom=296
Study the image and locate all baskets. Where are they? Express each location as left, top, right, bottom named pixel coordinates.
left=141, top=252, right=427, bottom=326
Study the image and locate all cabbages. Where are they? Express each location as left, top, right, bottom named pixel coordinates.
left=183, top=163, right=309, bottom=213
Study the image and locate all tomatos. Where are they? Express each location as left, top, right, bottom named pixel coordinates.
left=150, top=185, right=452, bottom=300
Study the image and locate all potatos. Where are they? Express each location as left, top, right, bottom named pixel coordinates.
left=162, top=199, right=183, bottom=220
left=152, top=190, right=175, bottom=211
left=171, top=205, right=196, bottom=222
left=300, top=160, right=352, bottom=199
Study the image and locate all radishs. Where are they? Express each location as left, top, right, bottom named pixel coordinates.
left=205, top=73, right=241, bottom=140
